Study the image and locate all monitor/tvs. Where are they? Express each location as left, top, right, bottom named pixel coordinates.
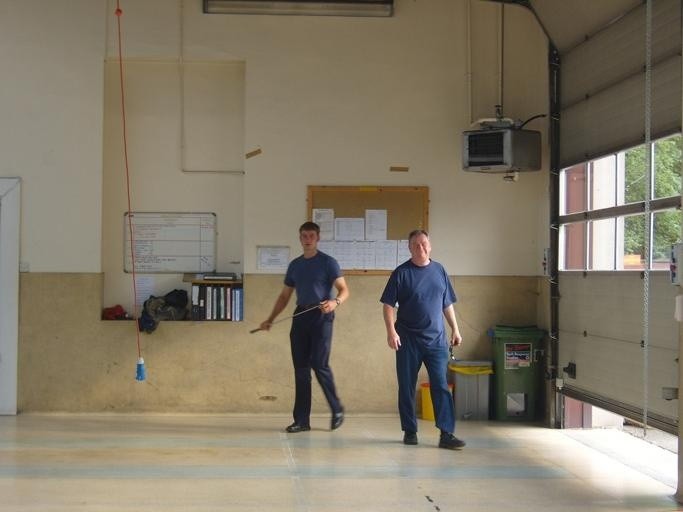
left=460, top=126, right=543, bottom=174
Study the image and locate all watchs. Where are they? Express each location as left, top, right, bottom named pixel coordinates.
left=334, top=297, right=341, bottom=305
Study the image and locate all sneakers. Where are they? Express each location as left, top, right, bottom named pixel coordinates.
left=286, top=421, right=311, bottom=432
left=332, top=405, right=344, bottom=429
left=440, top=433, right=466, bottom=448
left=404, top=431, right=418, bottom=445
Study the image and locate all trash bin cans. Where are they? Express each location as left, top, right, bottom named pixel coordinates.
left=486, top=324, right=548, bottom=422
left=449, top=358, right=493, bottom=422
left=420, top=383, right=455, bottom=421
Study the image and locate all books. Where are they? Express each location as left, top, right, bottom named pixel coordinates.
left=182, top=271, right=244, bottom=322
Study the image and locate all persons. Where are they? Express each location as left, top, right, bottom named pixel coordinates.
left=261, top=222, right=349, bottom=432
left=378, top=230, right=466, bottom=451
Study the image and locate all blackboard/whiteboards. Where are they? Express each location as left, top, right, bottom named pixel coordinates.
left=123, top=212, right=217, bottom=274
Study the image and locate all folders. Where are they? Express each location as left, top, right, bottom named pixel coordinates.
left=191, top=284, right=242, bottom=321
